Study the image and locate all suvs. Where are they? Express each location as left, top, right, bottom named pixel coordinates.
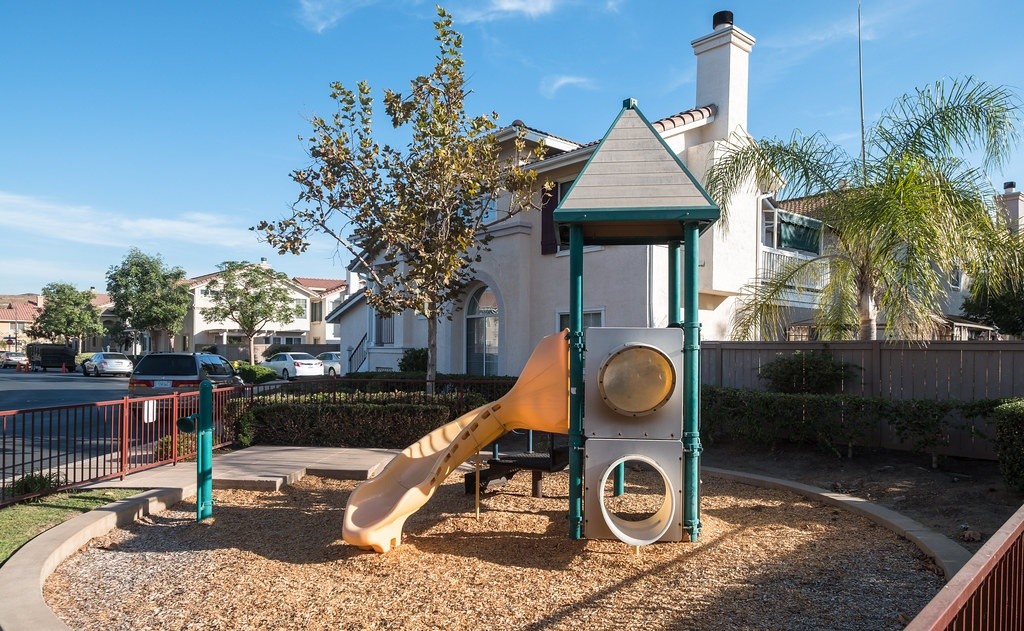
left=129, top=350, right=245, bottom=419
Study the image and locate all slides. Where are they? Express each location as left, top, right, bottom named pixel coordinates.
left=341, top=329, right=568, bottom=546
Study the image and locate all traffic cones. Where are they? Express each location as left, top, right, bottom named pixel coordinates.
left=60, top=363, right=67, bottom=373
left=23, top=363, right=29, bottom=372
left=15, top=361, right=21, bottom=372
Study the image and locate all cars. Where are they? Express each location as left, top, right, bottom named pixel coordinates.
left=0, top=351, right=29, bottom=369
left=262, top=352, right=324, bottom=381
left=83, top=352, right=134, bottom=378
left=316, top=351, right=341, bottom=378
